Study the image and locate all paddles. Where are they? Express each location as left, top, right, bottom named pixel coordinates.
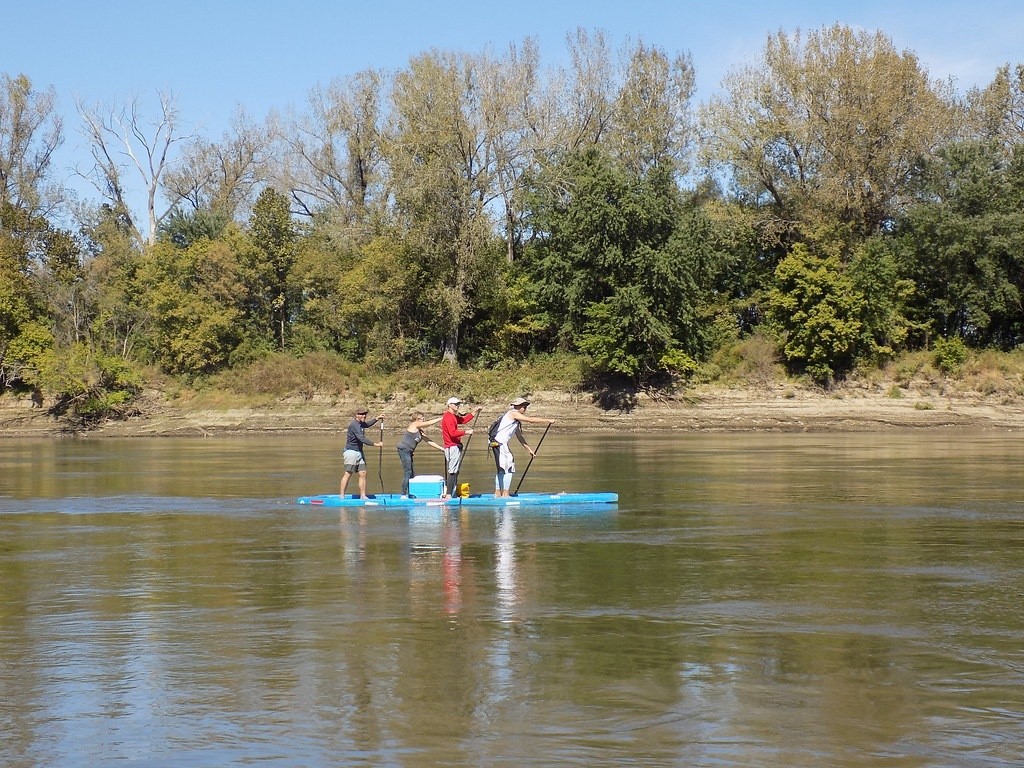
left=457, top=410, right=481, bottom=478
left=443, top=453, right=449, bottom=488
left=379, top=418, right=385, bottom=478
left=514, top=423, right=551, bottom=495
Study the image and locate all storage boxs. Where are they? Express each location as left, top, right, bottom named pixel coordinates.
left=409, top=474, right=445, bottom=499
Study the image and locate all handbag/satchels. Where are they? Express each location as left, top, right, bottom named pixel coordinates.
left=456, top=481, right=471, bottom=499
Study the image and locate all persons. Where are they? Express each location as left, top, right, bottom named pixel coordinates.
left=488, top=397, right=555, bottom=499
left=340, top=407, right=384, bottom=500
left=396, top=411, right=445, bottom=500
left=442, top=397, right=482, bottom=499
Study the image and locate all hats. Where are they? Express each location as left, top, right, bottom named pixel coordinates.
left=510, top=398, right=531, bottom=407
left=446, top=397, right=462, bottom=406
left=356, top=405, right=368, bottom=414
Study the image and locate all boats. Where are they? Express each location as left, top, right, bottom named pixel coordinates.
left=295, top=475, right=618, bottom=506
